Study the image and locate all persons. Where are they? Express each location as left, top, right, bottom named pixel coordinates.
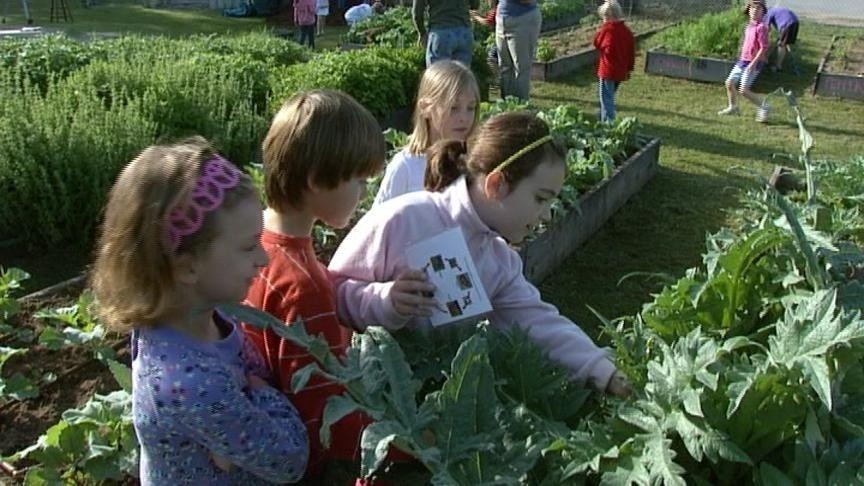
left=762, top=7, right=800, bottom=73
left=290, top=0, right=394, bottom=51
left=235, top=86, right=432, bottom=486
left=593, top=0, right=635, bottom=125
left=494, top=0, right=547, bottom=105
left=469, top=0, right=503, bottom=92
left=89, top=133, right=311, bottom=485
left=411, top=0, right=479, bottom=71
left=324, top=110, right=635, bottom=399
left=718, top=1, right=771, bottom=122
left=369, top=57, right=482, bottom=209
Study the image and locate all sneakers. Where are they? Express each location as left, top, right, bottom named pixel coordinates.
left=718, top=104, right=738, bottom=114
left=758, top=102, right=769, bottom=122
